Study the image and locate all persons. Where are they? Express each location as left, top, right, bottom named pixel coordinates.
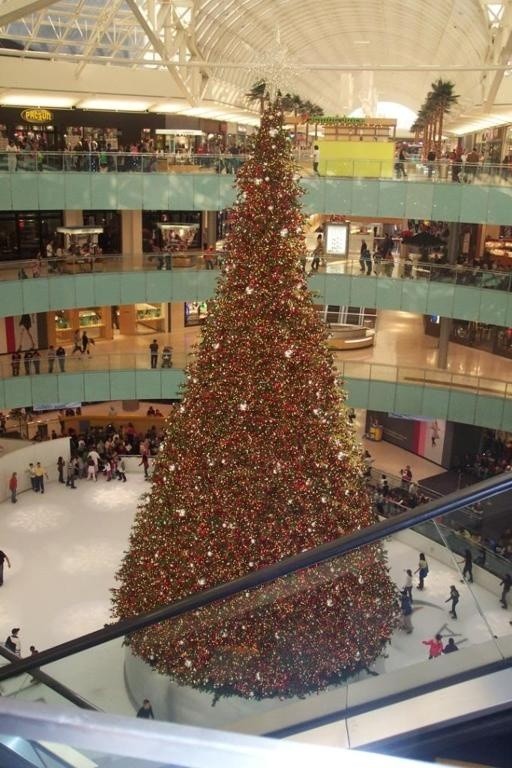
left=4, top=628, right=21, bottom=658
left=4, top=133, right=256, bottom=175
left=414, top=553, right=429, bottom=591
left=457, top=550, right=473, bottom=584
left=111, top=305, right=120, bottom=329
left=136, top=699, right=155, bottom=720
left=299, top=227, right=510, bottom=290
left=446, top=586, right=459, bottom=618
left=500, top=574, right=511, bottom=608
left=423, top=634, right=444, bottom=659
left=11, top=330, right=159, bottom=376
left=393, top=146, right=511, bottom=184
left=39, top=229, right=226, bottom=276
left=346, top=409, right=512, bottom=568
left=289, top=141, right=320, bottom=176
left=0, top=407, right=164, bottom=503
left=30, top=646, right=40, bottom=684
left=19, top=314, right=33, bottom=352
left=443, top=638, right=458, bottom=654
left=0, top=550, right=11, bottom=586
left=402, top=569, right=413, bottom=601
left=399, top=592, right=413, bottom=633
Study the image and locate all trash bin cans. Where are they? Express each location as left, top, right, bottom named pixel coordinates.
left=369, top=425, right=383, bottom=441
